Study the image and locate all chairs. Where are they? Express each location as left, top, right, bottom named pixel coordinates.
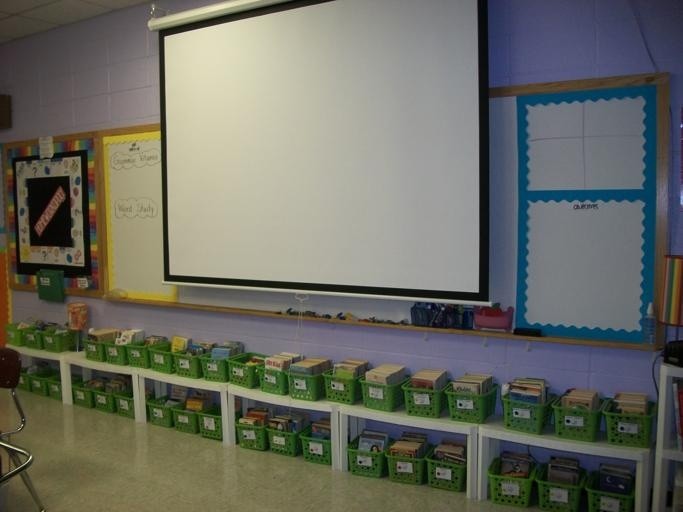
left=0, top=347, right=49, bottom=512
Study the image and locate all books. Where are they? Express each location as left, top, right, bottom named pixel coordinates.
left=356, top=427, right=467, bottom=465
left=496, top=450, right=636, bottom=496
left=80, top=371, right=130, bottom=394
left=672, top=378, right=683, bottom=451
left=245, top=351, right=495, bottom=397
left=86, top=325, right=245, bottom=359
left=508, top=376, right=648, bottom=417
left=163, top=383, right=214, bottom=412
left=14, top=316, right=73, bottom=377
left=238, top=406, right=330, bottom=439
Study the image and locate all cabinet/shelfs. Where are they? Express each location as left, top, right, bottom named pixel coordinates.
left=653, top=354, right=683, bottom=511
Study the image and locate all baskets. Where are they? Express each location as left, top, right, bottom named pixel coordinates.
left=5, top=323, right=225, bottom=441
left=500, top=392, right=658, bottom=448
left=486, top=456, right=635, bottom=512
left=226, top=353, right=498, bottom=492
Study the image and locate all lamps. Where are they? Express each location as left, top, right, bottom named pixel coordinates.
left=659, top=255, right=683, bottom=367
